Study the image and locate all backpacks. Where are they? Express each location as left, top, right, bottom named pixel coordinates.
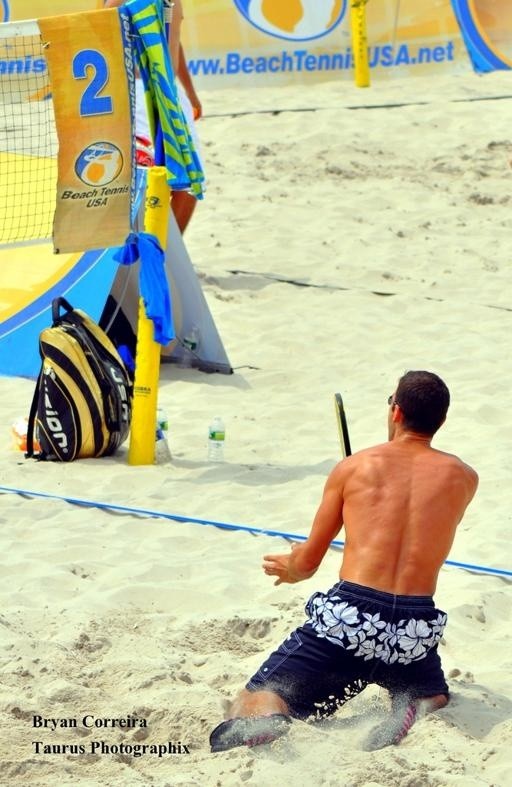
left=25, top=295, right=129, bottom=461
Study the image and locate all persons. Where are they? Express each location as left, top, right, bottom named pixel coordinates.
left=209, top=369, right=480, bottom=755
left=103, top=0, right=202, bottom=233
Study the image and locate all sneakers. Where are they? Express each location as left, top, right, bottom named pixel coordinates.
left=209, top=713, right=289, bottom=751
left=362, top=704, right=416, bottom=752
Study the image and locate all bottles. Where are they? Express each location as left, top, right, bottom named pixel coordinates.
left=206, top=415, right=226, bottom=463
left=175, top=326, right=199, bottom=368
left=155, top=408, right=172, bottom=467
